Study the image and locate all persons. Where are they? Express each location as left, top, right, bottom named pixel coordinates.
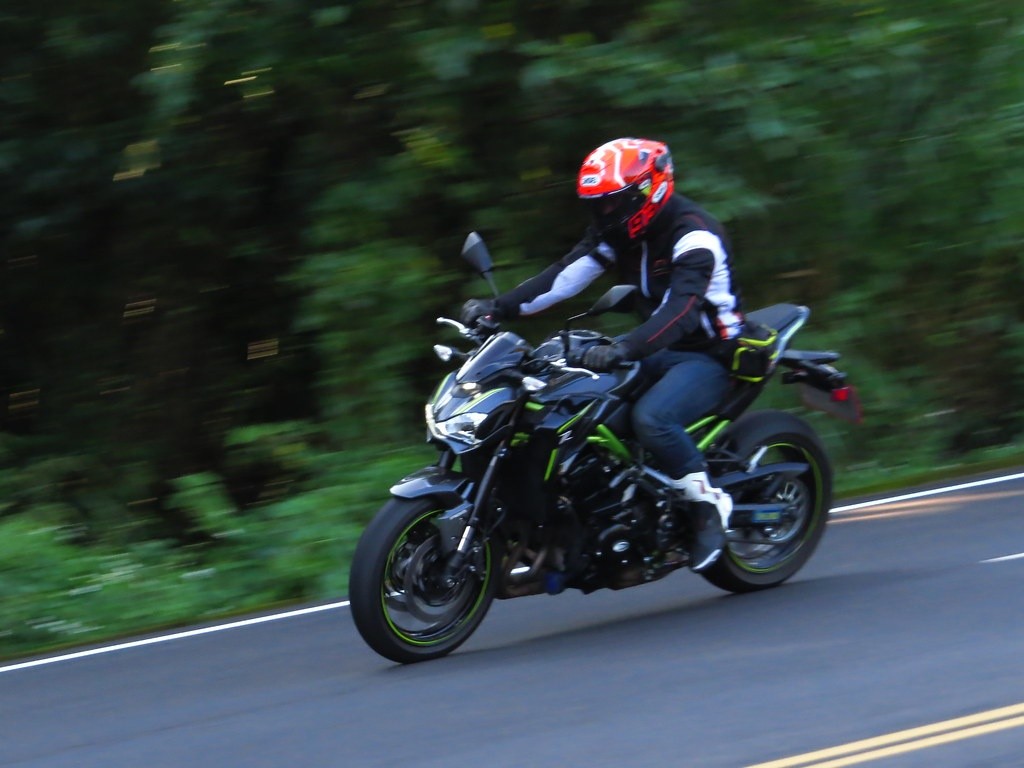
left=460, top=137, right=745, bottom=571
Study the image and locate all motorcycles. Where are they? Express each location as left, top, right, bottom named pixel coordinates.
left=347, top=229, right=855, bottom=668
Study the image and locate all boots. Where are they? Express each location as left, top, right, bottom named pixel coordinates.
left=670, top=471, right=733, bottom=572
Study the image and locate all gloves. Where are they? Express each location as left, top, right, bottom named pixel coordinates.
left=581, top=344, right=623, bottom=373
left=460, top=299, right=497, bottom=328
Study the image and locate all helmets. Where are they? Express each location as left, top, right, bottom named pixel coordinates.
left=577, top=138, right=674, bottom=241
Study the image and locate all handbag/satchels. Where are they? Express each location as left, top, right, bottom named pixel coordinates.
left=729, top=323, right=778, bottom=384
